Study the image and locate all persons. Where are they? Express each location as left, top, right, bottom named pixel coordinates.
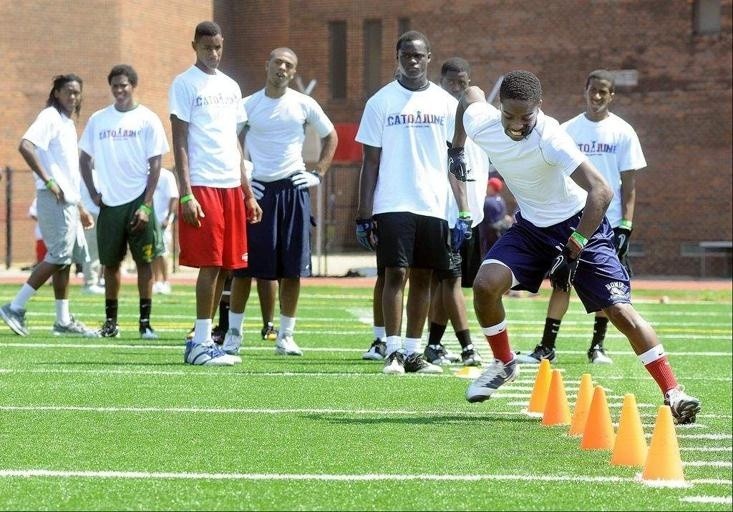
left=353, top=30, right=523, bottom=373
left=75, top=64, right=171, bottom=341
left=525, top=68, right=648, bottom=359
left=27, top=166, right=179, bottom=297
left=0, top=73, right=94, bottom=338
left=166, top=21, right=339, bottom=362
left=445, top=68, right=703, bottom=425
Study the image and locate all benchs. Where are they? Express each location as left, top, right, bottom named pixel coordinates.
left=698, top=240, right=732, bottom=278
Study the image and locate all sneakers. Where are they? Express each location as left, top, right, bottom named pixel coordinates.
left=587, top=342, right=614, bottom=365
left=466, top=349, right=521, bottom=404
left=383, top=352, right=405, bottom=374
left=52, top=314, right=92, bottom=337
left=140, top=322, right=158, bottom=340
left=461, top=343, right=483, bottom=366
left=663, top=385, right=701, bottom=425
left=151, top=281, right=172, bottom=296
left=516, top=346, right=557, bottom=365
left=183, top=326, right=243, bottom=365
left=0, top=304, right=30, bottom=338
left=424, top=345, right=460, bottom=365
left=363, top=341, right=385, bottom=360
left=276, top=335, right=303, bottom=356
left=262, top=322, right=279, bottom=340
left=84, top=320, right=121, bottom=339
left=406, top=353, right=444, bottom=374
left=80, top=283, right=107, bottom=296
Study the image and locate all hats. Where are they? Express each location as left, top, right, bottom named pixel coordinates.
left=488, top=177, right=502, bottom=193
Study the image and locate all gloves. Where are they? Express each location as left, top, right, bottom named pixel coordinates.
left=249, top=181, right=267, bottom=202
left=613, top=225, right=634, bottom=261
left=291, top=169, right=324, bottom=191
left=355, top=215, right=380, bottom=253
left=547, top=246, right=580, bottom=294
left=446, top=139, right=477, bottom=182
left=452, top=218, right=473, bottom=252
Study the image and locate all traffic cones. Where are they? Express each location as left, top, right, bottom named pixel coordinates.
left=523, top=356, right=690, bottom=484
